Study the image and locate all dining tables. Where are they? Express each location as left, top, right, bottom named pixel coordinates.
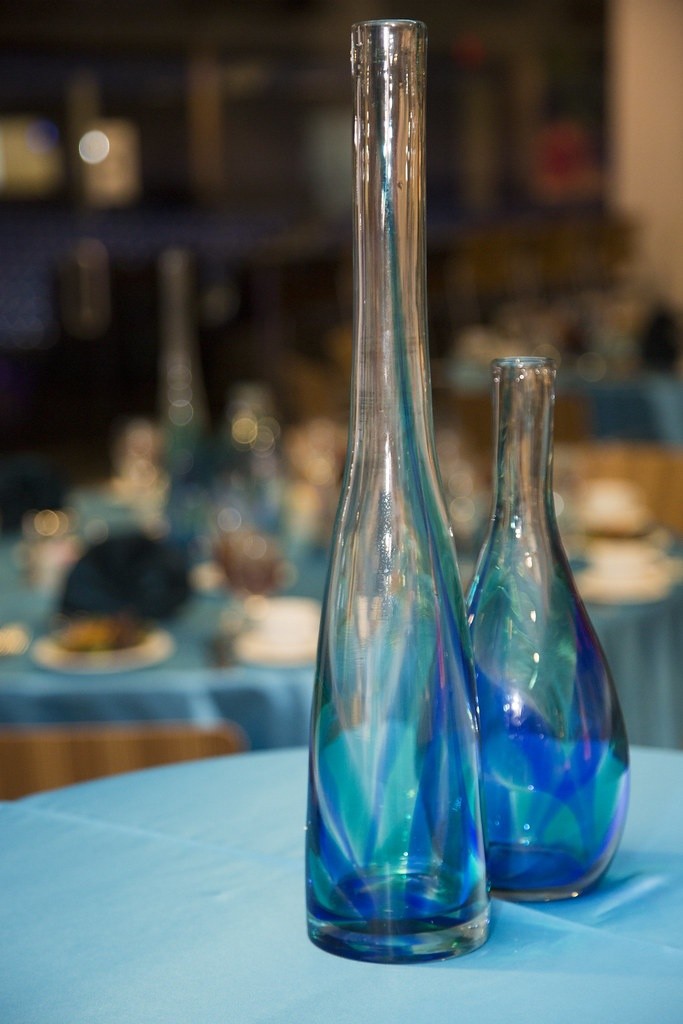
left=1, top=484, right=683, bottom=1024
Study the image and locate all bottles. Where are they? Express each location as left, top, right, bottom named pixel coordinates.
left=307, top=11, right=485, bottom=965
left=416, top=352, right=627, bottom=899
